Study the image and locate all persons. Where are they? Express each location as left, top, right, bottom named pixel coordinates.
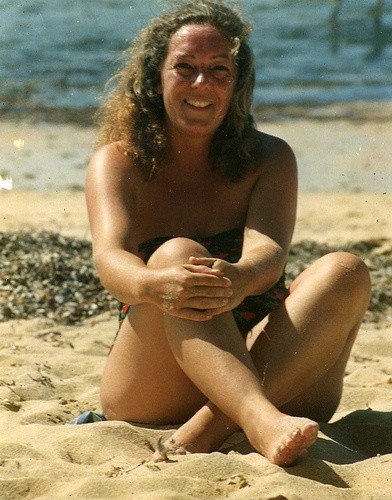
left=82, top=1, right=372, bottom=465
